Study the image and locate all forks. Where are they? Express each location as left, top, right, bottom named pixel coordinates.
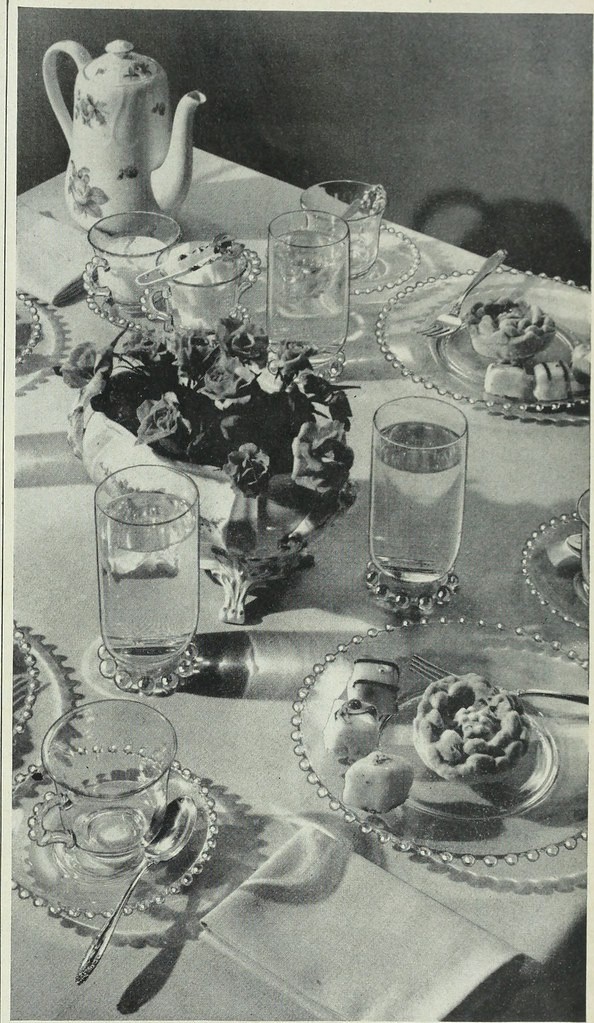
left=416, top=249, right=510, bottom=339
left=407, top=653, right=589, bottom=706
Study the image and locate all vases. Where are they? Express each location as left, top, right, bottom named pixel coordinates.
left=80, top=363, right=356, bottom=627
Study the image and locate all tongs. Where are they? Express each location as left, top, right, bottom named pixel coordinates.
left=134, top=233, right=245, bottom=288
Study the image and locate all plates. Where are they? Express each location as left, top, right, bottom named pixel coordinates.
left=15, top=291, right=41, bottom=366
left=13, top=623, right=40, bottom=758
left=11, top=750, right=219, bottom=918
left=521, top=510, right=590, bottom=630
left=290, top=618, right=589, bottom=867
left=87, top=294, right=168, bottom=332
left=266, top=226, right=592, bottom=425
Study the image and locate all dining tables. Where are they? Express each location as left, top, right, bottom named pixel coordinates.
left=11, top=144, right=591, bottom=1020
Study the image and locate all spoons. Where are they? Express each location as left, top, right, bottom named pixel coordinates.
left=74, top=794, right=200, bottom=986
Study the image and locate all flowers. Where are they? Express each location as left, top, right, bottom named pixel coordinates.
left=51, top=314, right=355, bottom=499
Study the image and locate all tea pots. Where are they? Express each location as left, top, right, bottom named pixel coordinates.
left=41, top=38, right=207, bottom=233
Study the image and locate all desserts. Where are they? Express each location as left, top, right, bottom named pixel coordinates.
left=465, top=297, right=593, bottom=402
left=414, top=674, right=524, bottom=777
left=344, top=751, right=414, bottom=812
left=351, top=657, right=398, bottom=712
left=326, top=699, right=380, bottom=761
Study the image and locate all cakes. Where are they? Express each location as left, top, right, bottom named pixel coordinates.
left=59, top=308, right=356, bottom=528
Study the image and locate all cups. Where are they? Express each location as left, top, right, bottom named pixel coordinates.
left=87, top=210, right=261, bottom=332
left=28, top=697, right=178, bottom=868
left=94, top=465, right=202, bottom=697
left=363, top=395, right=467, bottom=617
left=268, top=179, right=386, bottom=380
left=566, top=489, right=590, bottom=606
